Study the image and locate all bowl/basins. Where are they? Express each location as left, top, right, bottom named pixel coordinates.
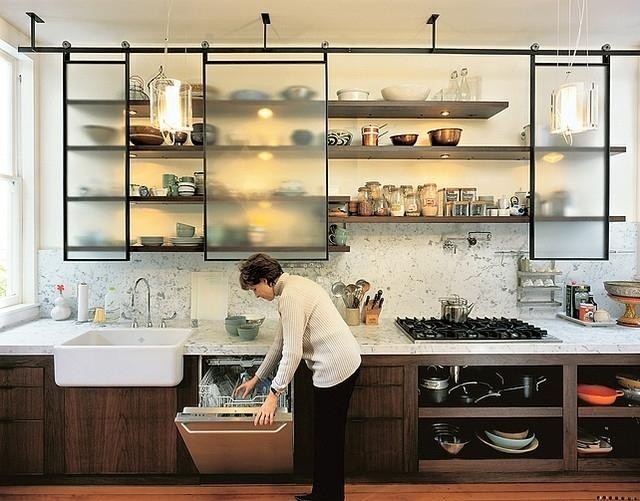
left=170, top=131, right=188, bottom=145
left=141, top=223, right=195, bottom=247
left=190, top=122, right=220, bottom=145
left=130, top=125, right=168, bottom=145
left=224, top=314, right=265, bottom=342
left=426, top=129, right=462, bottom=148
left=178, top=84, right=218, bottom=101
left=390, top=132, right=418, bottom=146
left=361, top=122, right=389, bottom=146
left=323, top=130, right=353, bottom=146
left=336, top=89, right=369, bottom=101
left=81, top=122, right=120, bottom=144
left=604, top=281, right=640, bottom=296
left=381, top=86, right=430, bottom=101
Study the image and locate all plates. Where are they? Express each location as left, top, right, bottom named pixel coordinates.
left=170, top=236, right=203, bottom=249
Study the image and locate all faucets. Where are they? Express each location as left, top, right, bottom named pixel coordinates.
left=130, top=278, right=154, bottom=327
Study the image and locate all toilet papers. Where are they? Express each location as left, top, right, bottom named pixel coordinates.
left=77, top=283, right=89, bottom=322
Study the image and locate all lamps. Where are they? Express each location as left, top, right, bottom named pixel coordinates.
left=549, top=1, right=598, bottom=146
left=146, top=0, right=195, bottom=146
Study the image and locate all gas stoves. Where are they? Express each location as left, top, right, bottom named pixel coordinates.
left=395, top=316, right=563, bottom=345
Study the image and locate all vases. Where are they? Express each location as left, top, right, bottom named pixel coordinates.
left=51, top=293, right=71, bottom=321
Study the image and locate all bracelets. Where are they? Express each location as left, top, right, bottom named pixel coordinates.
left=271, top=387, right=281, bottom=397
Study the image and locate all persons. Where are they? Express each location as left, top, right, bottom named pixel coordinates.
left=234, top=253, right=363, bottom=501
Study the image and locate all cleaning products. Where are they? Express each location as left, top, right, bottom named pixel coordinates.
left=106, top=286, right=120, bottom=323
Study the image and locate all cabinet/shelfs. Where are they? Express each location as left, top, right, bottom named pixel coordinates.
left=65, top=99, right=626, bottom=253
left=563, top=354, right=640, bottom=482
left=0, top=355, right=53, bottom=487
left=298, top=354, right=418, bottom=485
left=516, top=260, right=562, bottom=313
left=404, top=355, right=577, bottom=483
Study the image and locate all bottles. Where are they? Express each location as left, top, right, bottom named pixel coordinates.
left=459, top=76, right=471, bottom=102
left=103, top=285, right=121, bottom=328
left=575, top=284, right=589, bottom=319
left=348, top=181, right=460, bottom=218
left=586, top=293, right=598, bottom=313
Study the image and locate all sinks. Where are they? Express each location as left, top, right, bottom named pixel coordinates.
left=55, top=328, right=192, bottom=386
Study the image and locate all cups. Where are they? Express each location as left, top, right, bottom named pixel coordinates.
left=470, top=75, right=482, bottom=100
left=129, top=172, right=206, bottom=198
left=486, top=187, right=569, bottom=220
left=593, top=311, right=612, bottom=325
left=516, top=258, right=566, bottom=289
left=328, top=223, right=348, bottom=248
left=94, top=306, right=106, bottom=325
left=206, top=367, right=290, bottom=410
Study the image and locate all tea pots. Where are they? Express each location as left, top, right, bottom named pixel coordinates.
left=437, top=294, right=476, bottom=324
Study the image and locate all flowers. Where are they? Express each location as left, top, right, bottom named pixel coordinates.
left=57, top=284, right=64, bottom=293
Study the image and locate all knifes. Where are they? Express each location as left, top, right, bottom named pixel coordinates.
left=364, top=289, right=385, bottom=309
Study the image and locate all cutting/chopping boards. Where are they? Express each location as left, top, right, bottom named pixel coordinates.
left=189, top=269, right=229, bottom=321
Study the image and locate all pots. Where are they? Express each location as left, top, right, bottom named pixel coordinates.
left=420, top=364, right=639, bottom=460
left=518, top=123, right=557, bottom=151
